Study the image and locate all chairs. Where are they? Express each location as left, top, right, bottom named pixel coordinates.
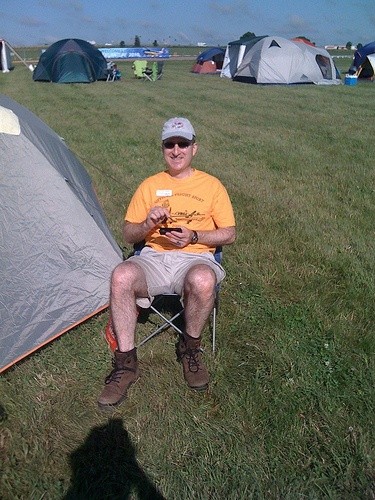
left=126, top=239, right=222, bottom=356
left=131, top=60, right=164, bottom=82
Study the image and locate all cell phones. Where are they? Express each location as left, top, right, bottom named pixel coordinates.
left=159, top=227, right=182, bottom=235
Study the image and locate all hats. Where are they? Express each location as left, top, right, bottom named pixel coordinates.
left=161, top=117, right=196, bottom=141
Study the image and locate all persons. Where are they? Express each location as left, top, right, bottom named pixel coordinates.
left=98, top=117, right=237, bottom=405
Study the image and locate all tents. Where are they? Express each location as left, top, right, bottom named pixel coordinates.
left=347, top=41, right=375, bottom=80
left=0, top=91, right=122, bottom=368
left=32, top=38, right=120, bottom=82
left=192, top=35, right=342, bottom=86
left=0, top=38, right=29, bottom=70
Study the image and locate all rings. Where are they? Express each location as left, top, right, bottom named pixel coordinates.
left=177, top=241, right=179, bottom=244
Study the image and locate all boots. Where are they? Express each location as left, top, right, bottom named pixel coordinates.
left=97, top=347, right=138, bottom=407
left=176, top=331, right=209, bottom=390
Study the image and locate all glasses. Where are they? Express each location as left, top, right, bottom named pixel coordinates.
left=163, top=142, right=190, bottom=149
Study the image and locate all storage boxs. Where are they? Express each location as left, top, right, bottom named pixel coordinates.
left=345, top=74, right=358, bottom=85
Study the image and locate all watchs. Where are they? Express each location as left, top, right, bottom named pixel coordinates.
left=192, top=230, right=198, bottom=244
